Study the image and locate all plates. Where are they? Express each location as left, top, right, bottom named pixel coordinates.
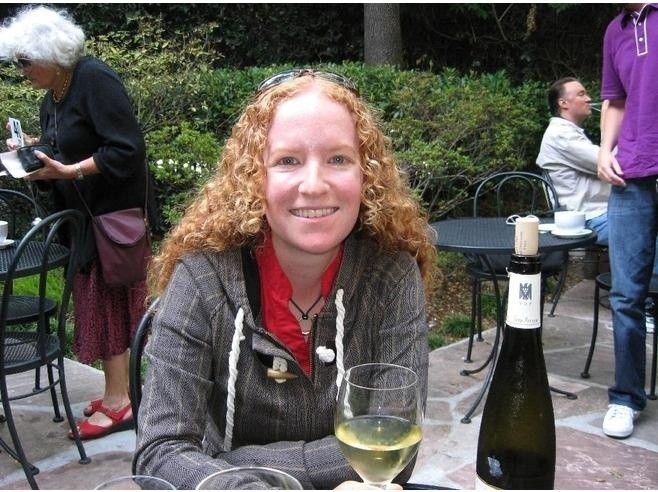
left=551, top=228, right=594, bottom=239
left=0, top=239, right=15, bottom=250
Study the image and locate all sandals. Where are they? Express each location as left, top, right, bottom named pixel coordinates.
left=68, top=404, right=136, bottom=440
left=84, top=399, right=102, bottom=415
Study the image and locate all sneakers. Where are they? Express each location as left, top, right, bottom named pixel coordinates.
left=603, top=402, right=642, bottom=438
left=646, top=316, right=654, bottom=333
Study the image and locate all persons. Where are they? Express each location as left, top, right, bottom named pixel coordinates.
left=535, top=77, right=657, bottom=314
left=130, top=67, right=445, bottom=491
left=0, top=3, right=169, bottom=439
left=595, top=3, right=658, bottom=439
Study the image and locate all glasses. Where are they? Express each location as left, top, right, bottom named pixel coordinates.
left=257, top=68, right=360, bottom=92
left=11, top=58, right=33, bottom=68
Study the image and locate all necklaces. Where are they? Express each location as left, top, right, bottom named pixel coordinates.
left=285, top=291, right=323, bottom=336
left=52, top=71, right=70, bottom=104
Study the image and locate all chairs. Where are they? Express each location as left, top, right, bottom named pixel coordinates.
left=1, top=208, right=91, bottom=491
left=129, top=292, right=162, bottom=437
left=579, top=270, right=657, bottom=401
left=462, top=171, right=558, bottom=364
left=539, top=168, right=609, bottom=317
left=0, top=188, right=58, bottom=391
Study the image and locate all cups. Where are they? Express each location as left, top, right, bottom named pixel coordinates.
left=96, top=476, right=177, bottom=490
left=0, top=220, right=8, bottom=243
left=197, top=469, right=305, bottom=489
left=553, top=211, right=585, bottom=234
left=332, top=362, right=425, bottom=487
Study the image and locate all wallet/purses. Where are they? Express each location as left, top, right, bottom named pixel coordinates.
left=19, top=145, right=53, bottom=172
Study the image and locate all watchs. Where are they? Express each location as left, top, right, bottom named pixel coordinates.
left=74, top=162, right=84, bottom=181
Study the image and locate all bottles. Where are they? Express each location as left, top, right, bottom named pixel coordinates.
left=474, top=215, right=554, bottom=490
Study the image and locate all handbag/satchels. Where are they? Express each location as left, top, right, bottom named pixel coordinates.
left=72, top=157, right=153, bottom=287
